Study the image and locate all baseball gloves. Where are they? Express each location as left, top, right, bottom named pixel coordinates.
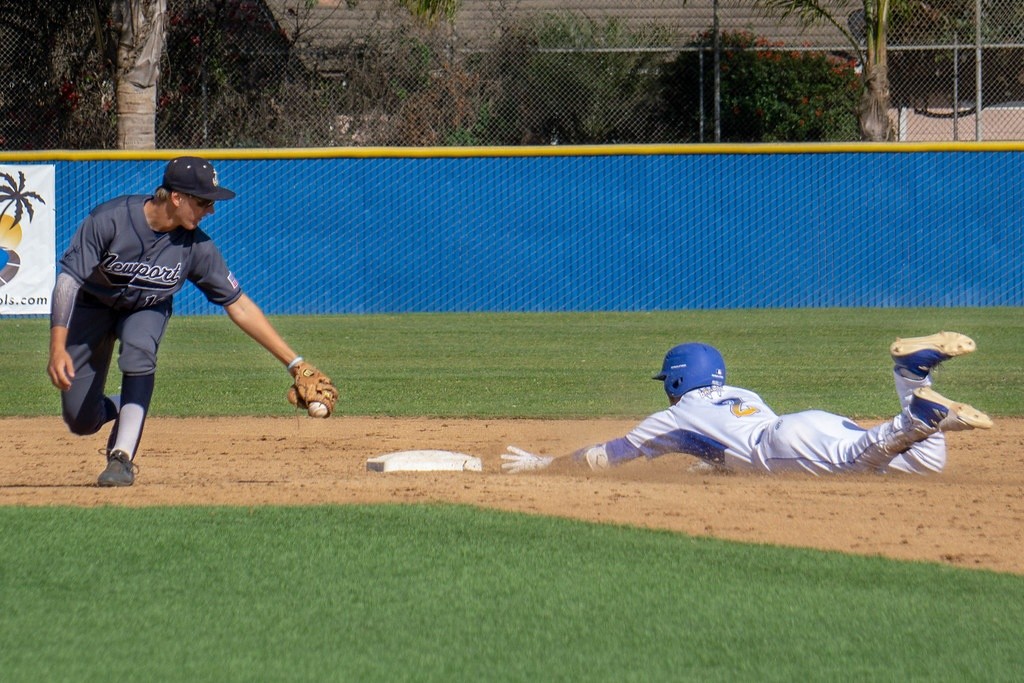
left=286, top=356, right=340, bottom=430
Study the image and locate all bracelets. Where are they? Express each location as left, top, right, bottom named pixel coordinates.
left=287, top=357, right=302, bottom=371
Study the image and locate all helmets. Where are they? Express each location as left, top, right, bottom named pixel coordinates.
left=653, top=343, right=726, bottom=397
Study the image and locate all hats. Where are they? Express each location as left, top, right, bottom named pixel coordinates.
left=164, top=157, right=236, bottom=201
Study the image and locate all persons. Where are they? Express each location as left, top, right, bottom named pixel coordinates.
left=501, top=332, right=992, bottom=473
left=46, top=156, right=340, bottom=486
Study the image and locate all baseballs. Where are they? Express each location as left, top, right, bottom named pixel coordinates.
left=308, top=401, right=328, bottom=418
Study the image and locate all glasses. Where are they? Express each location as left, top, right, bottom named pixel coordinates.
left=189, top=194, right=214, bottom=208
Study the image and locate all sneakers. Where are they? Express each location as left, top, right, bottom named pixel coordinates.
left=99, top=448, right=140, bottom=486
left=890, top=330, right=977, bottom=378
left=107, top=418, right=120, bottom=454
left=909, top=387, right=993, bottom=431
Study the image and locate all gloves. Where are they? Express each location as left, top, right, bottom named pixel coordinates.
left=501, top=446, right=552, bottom=475
